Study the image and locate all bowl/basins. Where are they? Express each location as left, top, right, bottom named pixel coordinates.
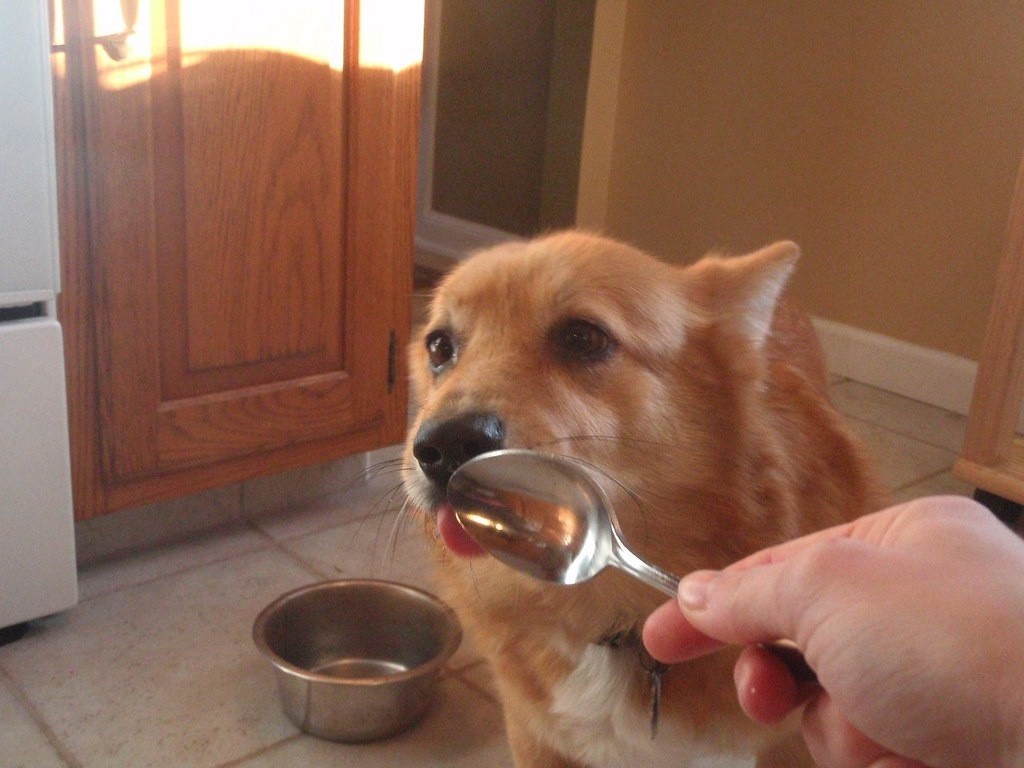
left=249, top=578, right=465, bottom=747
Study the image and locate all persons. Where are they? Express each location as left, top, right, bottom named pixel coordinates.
left=639, top=494, right=1024, bottom=768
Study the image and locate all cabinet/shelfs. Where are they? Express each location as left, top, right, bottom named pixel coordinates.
left=52, top=0, right=432, bottom=518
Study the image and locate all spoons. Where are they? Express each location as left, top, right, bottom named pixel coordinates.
left=446, top=451, right=817, bottom=686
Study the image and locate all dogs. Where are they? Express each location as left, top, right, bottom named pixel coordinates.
left=398, top=232, right=879, bottom=768
